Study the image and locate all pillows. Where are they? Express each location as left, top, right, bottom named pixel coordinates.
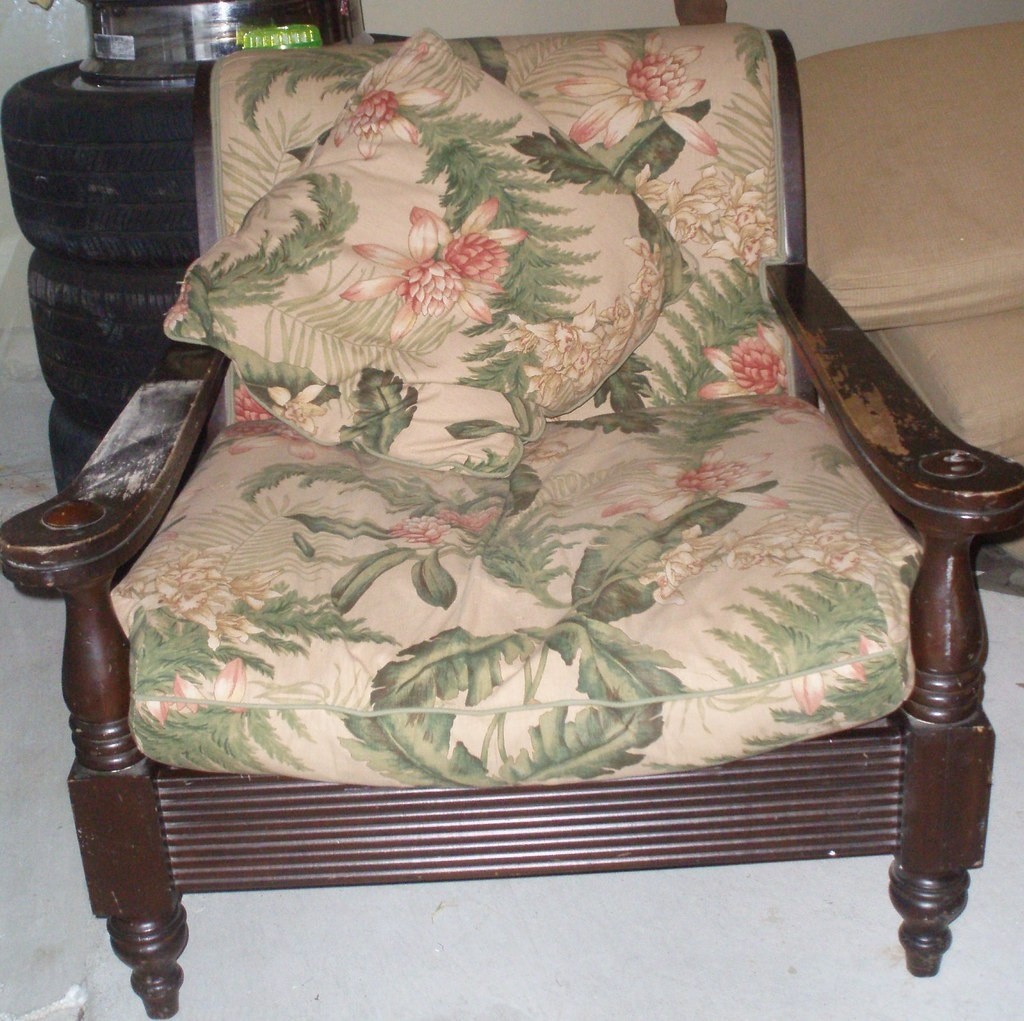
left=163, top=27, right=699, bottom=481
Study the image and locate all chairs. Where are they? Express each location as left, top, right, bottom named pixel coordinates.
left=0, top=22, right=1024, bottom=1020
